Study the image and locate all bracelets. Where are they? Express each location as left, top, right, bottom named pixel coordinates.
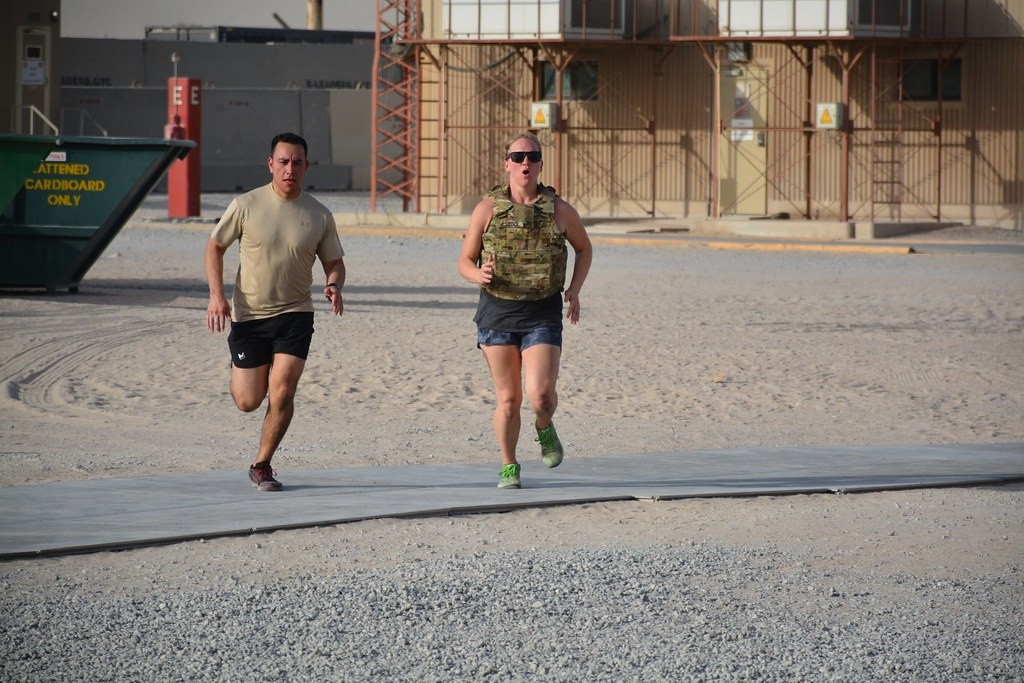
left=325, top=283, right=341, bottom=292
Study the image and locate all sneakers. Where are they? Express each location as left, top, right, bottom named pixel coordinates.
left=498, top=464, right=521, bottom=488
left=249, top=462, right=282, bottom=491
left=536, top=418, right=563, bottom=468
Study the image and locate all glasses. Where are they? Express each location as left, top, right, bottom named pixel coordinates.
left=507, top=151, right=542, bottom=163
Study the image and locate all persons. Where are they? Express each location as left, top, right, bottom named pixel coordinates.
left=458, top=133, right=593, bottom=489
left=204, top=132, right=346, bottom=492
